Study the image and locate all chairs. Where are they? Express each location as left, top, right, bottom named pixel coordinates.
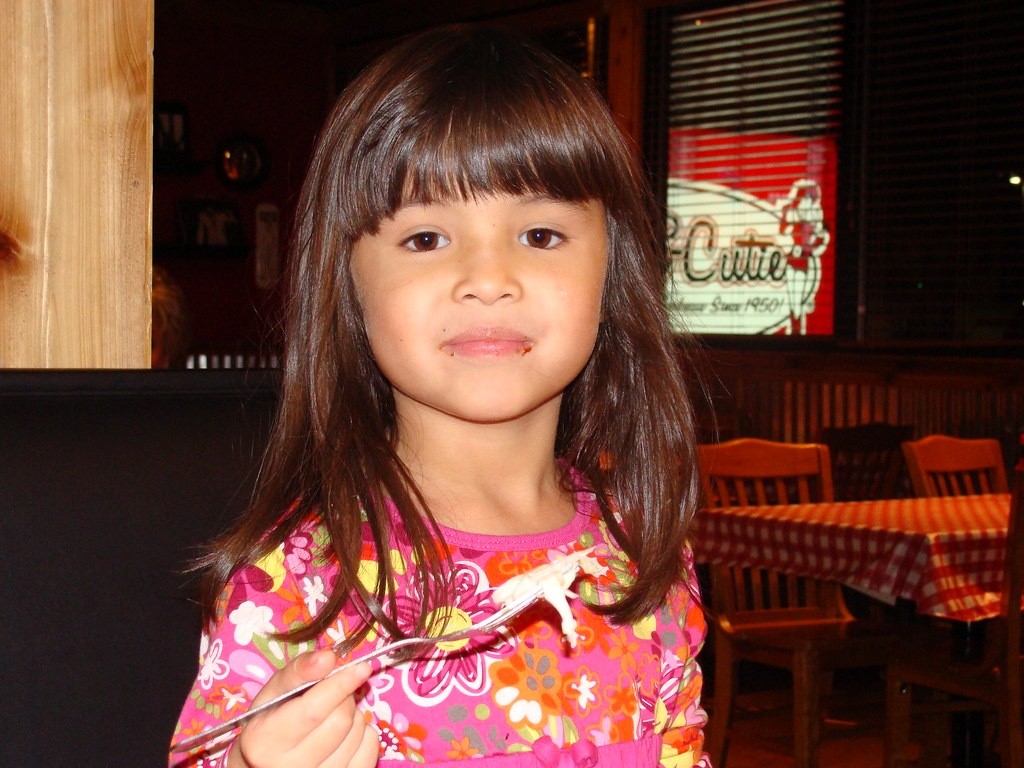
left=696, top=435, right=1024, bottom=768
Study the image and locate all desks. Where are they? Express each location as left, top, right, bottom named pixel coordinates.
left=687, top=494, right=1024, bottom=744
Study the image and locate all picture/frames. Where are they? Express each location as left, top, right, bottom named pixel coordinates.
left=174, top=197, right=244, bottom=249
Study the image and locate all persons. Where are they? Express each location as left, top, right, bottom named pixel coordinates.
left=168, top=23, right=714, bottom=768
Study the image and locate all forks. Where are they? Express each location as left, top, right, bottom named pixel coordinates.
left=169, top=560, right=581, bottom=754
